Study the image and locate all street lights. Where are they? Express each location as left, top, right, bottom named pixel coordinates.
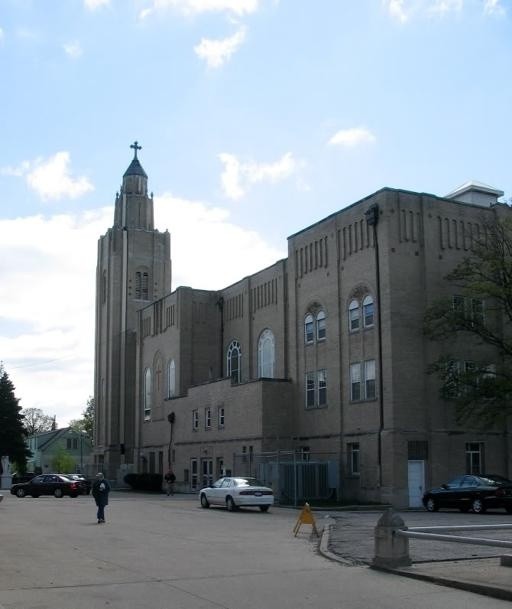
left=79, top=429, right=88, bottom=471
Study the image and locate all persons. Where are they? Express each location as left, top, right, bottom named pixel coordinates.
left=164, top=467, right=176, bottom=497
left=92, top=472, right=111, bottom=524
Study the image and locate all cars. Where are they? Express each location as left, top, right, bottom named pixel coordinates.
left=199, top=476, right=274, bottom=514
left=421, top=474, right=512, bottom=516
left=9, top=472, right=93, bottom=499
